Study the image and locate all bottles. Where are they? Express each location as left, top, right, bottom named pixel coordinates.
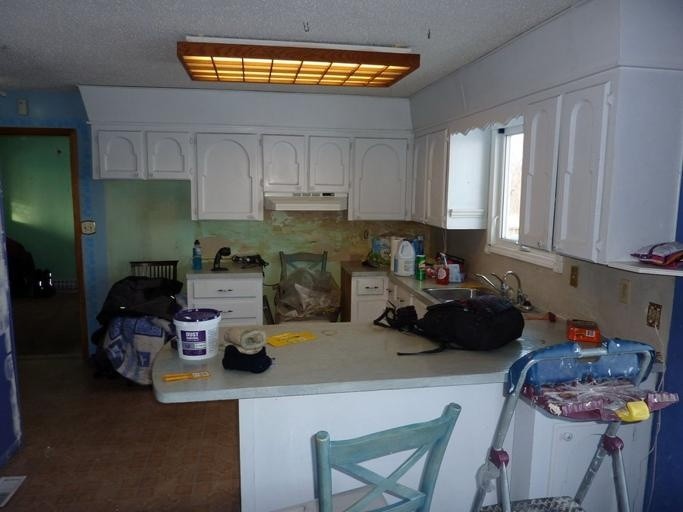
left=192, top=240, right=202, bottom=270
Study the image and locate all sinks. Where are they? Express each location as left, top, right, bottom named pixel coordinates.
left=422, top=286, right=499, bottom=303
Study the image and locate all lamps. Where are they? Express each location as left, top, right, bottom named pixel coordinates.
left=176, top=35, right=420, bottom=87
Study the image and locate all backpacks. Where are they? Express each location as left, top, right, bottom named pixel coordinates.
left=374, top=290, right=526, bottom=356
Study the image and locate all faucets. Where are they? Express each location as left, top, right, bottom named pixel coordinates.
left=471, top=273, right=502, bottom=292
left=502, top=270, right=524, bottom=304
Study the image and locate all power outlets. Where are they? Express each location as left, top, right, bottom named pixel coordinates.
left=647, top=302, right=663, bottom=328
left=81, top=220, right=96, bottom=234
left=570, top=266, right=578, bottom=287
left=620, top=279, right=631, bottom=303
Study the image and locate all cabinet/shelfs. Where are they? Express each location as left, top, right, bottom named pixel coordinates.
left=412, top=123, right=491, bottom=229
left=88, top=121, right=193, bottom=181
left=348, top=129, right=415, bottom=222
left=412, top=289, right=436, bottom=320
left=259, top=126, right=350, bottom=212
left=187, top=258, right=264, bottom=326
left=341, top=260, right=387, bottom=321
left=194, top=125, right=265, bottom=221
left=388, top=277, right=412, bottom=326
left=519, top=66, right=682, bottom=262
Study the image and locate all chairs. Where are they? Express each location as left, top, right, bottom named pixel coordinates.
left=128, top=260, right=180, bottom=282
left=268, top=403, right=461, bottom=512
left=468, top=338, right=678, bottom=512
left=278, top=249, right=328, bottom=284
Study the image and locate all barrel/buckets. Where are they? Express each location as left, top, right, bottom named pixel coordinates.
left=393, top=238, right=417, bottom=277
left=172, top=308, right=222, bottom=361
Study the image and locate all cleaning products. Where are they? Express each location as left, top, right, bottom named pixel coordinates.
left=437, top=252, right=450, bottom=285
